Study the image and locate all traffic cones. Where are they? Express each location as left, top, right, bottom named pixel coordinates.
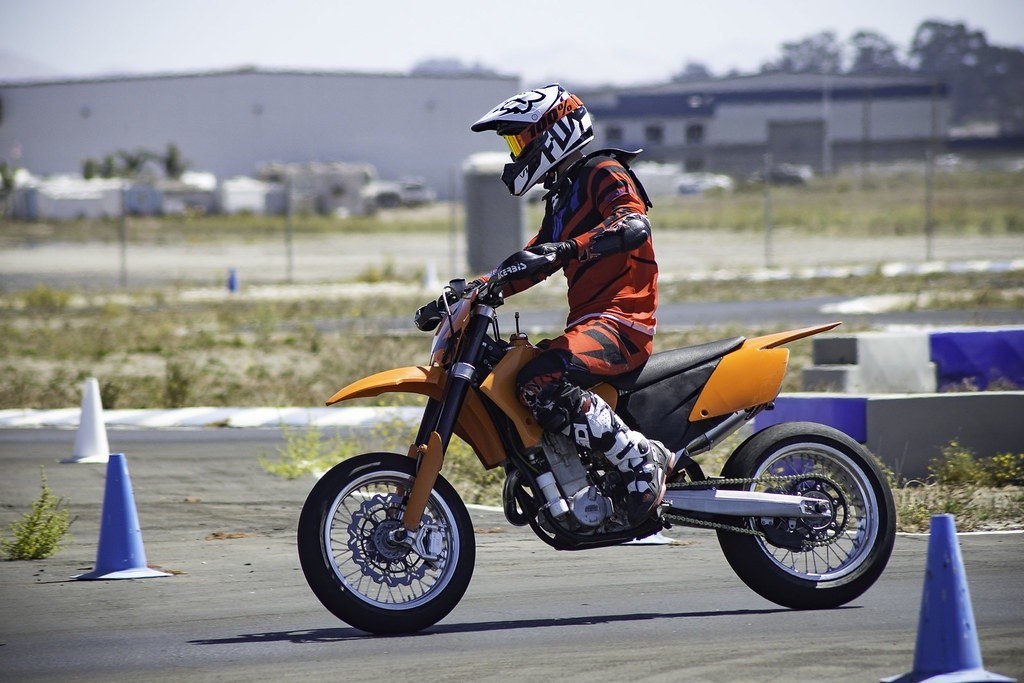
left=69, top=453, right=177, bottom=581
left=59, top=377, right=110, bottom=466
left=874, top=511, right=1018, bottom=682
left=225, top=268, right=241, bottom=293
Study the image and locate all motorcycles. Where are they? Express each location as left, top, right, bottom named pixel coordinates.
left=296, top=240, right=897, bottom=638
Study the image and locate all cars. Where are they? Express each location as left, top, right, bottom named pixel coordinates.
left=750, top=160, right=815, bottom=189
left=678, top=171, right=732, bottom=193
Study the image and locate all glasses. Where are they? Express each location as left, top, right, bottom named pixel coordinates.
left=502, top=134, right=526, bottom=159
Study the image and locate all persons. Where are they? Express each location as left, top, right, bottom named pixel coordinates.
left=435, top=83, right=675, bottom=527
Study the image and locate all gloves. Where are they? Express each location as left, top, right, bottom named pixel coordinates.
left=540, top=241, right=577, bottom=261
left=438, top=280, right=478, bottom=309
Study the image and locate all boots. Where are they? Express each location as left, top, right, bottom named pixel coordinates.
left=558, top=392, right=674, bottom=525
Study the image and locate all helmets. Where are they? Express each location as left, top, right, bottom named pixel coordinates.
left=471, top=85, right=594, bottom=197
